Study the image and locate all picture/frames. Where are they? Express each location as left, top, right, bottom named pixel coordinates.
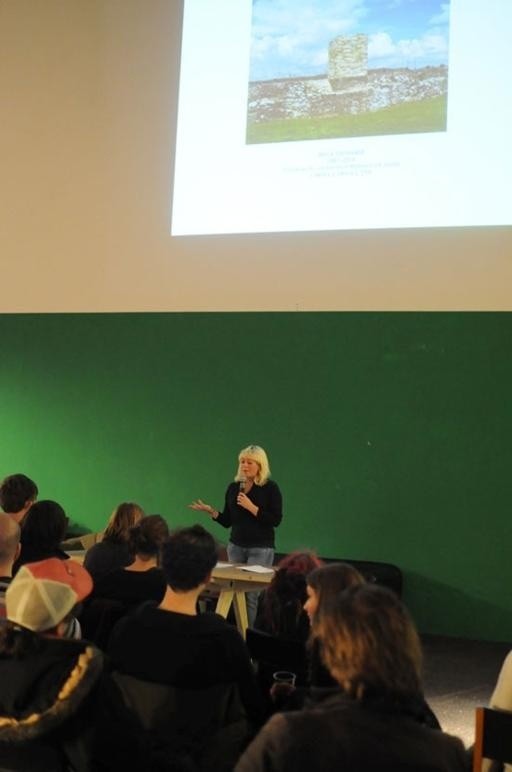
left=64, top=551, right=279, bottom=643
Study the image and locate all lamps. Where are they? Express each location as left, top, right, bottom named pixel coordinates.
left=472, top=707, right=512, bottom=772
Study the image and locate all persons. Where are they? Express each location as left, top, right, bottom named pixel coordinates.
left=11, top=500, right=69, bottom=578
left=187, top=445, right=283, bottom=630
left=117, top=514, right=170, bottom=608
left=2, top=473, right=39, bottom=521
left=82, top=502, right=145, bottom=600
left=270, top=562, right=365, bottom=711
left=0, top=514, right=21, bottom=598
left=253, top=551, right=323, bottom=634
left=107, top=525, right=297, bottom=725
left=234, top=581, right=473, bottom=770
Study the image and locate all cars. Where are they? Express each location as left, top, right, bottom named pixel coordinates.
left=272, top=671, right=297, bottom=691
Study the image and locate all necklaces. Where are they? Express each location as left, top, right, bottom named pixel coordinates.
left=1, top=559, right=148, bottom=771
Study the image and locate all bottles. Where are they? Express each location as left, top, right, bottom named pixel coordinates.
left=5, top=558, right=93, bottom=633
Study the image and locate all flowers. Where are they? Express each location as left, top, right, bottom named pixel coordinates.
left=238, top=476, right=247, bottom=513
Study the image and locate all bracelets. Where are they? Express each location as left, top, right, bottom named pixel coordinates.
left=208, top=510, right=215, bottom=514
